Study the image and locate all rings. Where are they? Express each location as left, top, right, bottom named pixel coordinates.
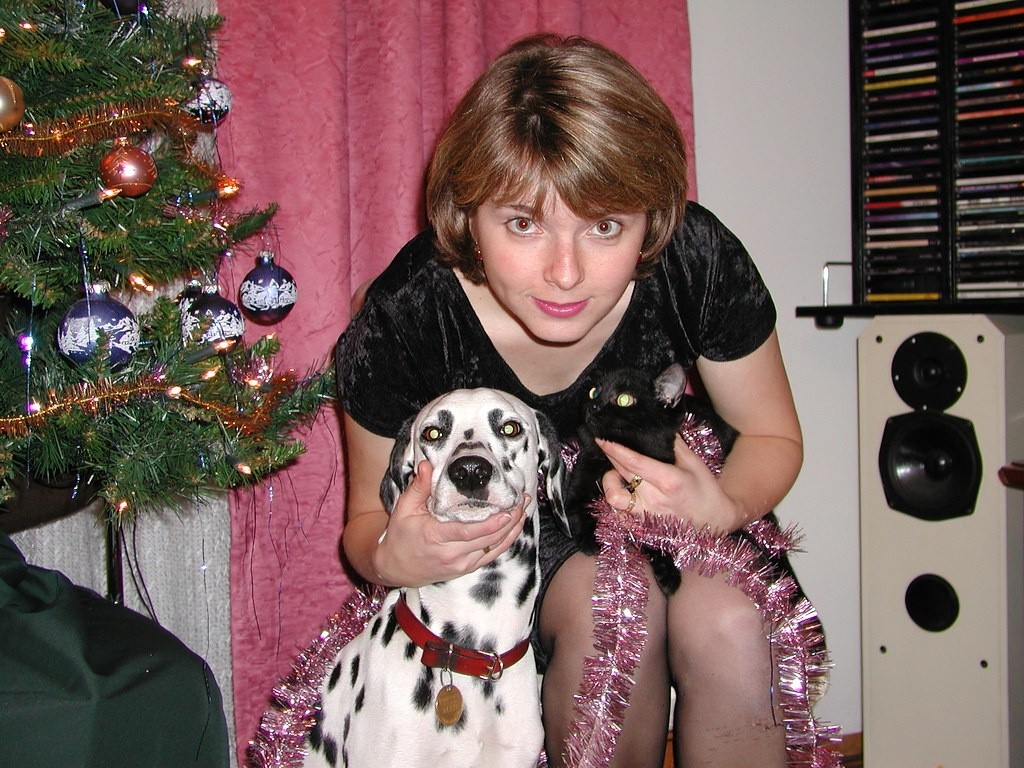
left=624, top=493, right=637, bottom=513
left=630, top=475, right=643, bottom=488
left=483, top=546, right=491, bottom=553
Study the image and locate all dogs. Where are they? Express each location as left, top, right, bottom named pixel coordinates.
left=300, top=388, right=574, bottom=768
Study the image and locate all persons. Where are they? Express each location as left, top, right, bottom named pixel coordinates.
left=330, top=33, right=804, bottom=768
